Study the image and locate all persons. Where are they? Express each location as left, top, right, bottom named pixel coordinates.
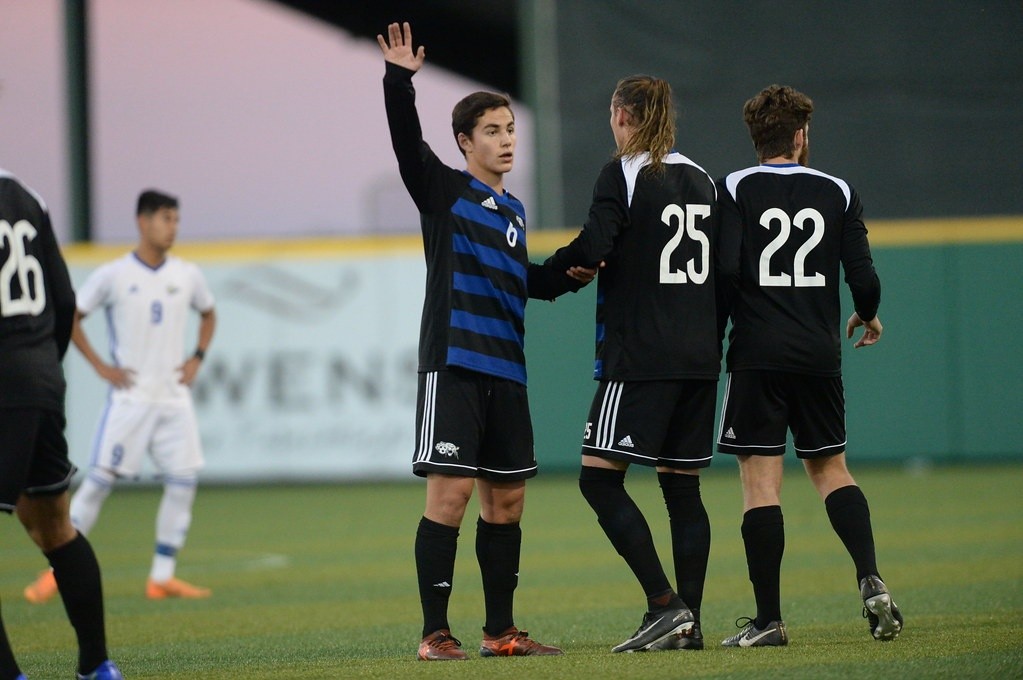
left=0, top=174, right=124, bottom=680
left=376, top=20, right=606, bottom=662
left=543, top=72, right=718, bottom=653
left=714, top=84, right=904, bottom=646
left=23, top=187, right=217, bottom=608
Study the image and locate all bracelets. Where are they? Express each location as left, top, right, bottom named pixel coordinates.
left=195, top=350, right=204, bottom=359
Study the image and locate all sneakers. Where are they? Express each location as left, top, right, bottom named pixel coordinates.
left=860, top=575, right=903, bottom=641
left=417, top=629, right=471, bottom=660
left=76, top=660, right=122, bottom=680
left=23, top=569, right=58, bottom=602
left=721, top=616, right=788, bottom=647
left=16, top=672, right=28, bottom=680
left=145, top=574, right=209, bottom=599
left=636, top=610, right=703, bottom=650
left=610, top=593, right=694, bottom=654
left=480, top=626, right=563, bottom=657
